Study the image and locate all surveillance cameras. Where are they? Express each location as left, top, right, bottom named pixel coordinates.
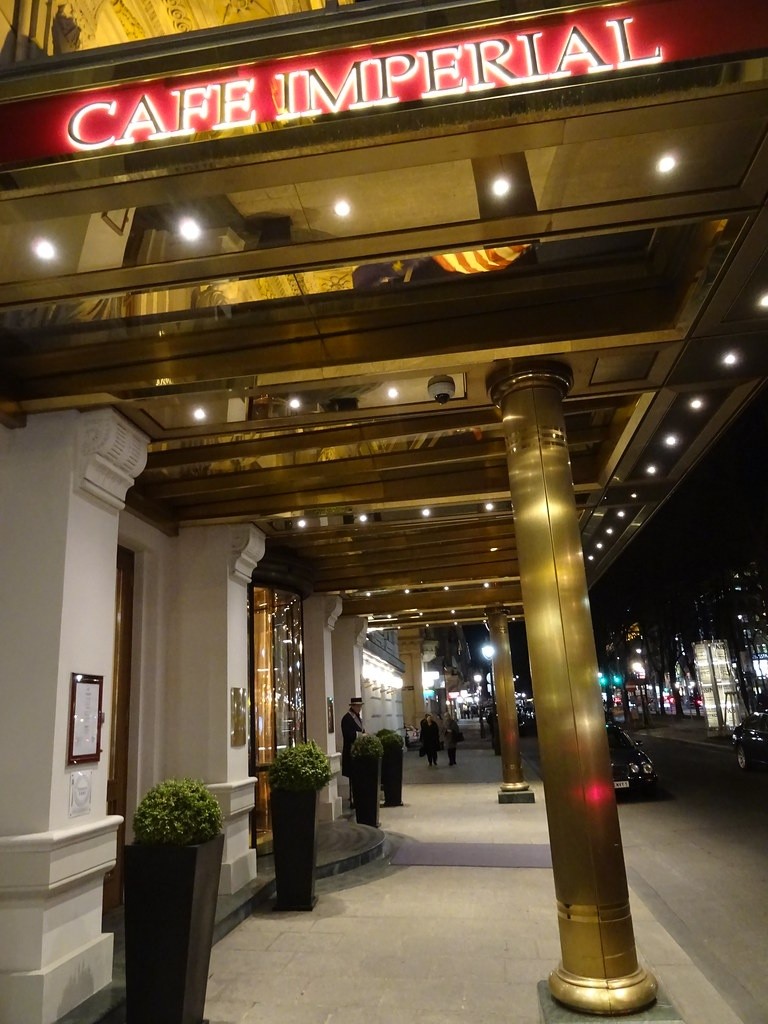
left=426, top=375, right=456, bottom=405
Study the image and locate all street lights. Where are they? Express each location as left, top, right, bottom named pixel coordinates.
left=478, top=641, right=502, bottom=756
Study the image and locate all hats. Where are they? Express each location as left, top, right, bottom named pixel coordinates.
left=349, top=697, right=363, bottom=706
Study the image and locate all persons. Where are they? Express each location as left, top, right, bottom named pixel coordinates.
left=420, top=704, right=499, bottom=767
left=341, top=698, right=366, bottom=809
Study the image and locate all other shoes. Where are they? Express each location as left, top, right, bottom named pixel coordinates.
left=434, top=762, right=437, bottom=765
left=449, top=763, right=453, bottom=766
left=454, top=761, right=456, bottom=764
left=429, top=762, right=432, bottom=766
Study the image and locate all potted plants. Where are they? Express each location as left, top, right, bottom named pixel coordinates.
left=352, top=735, right=382, bottom=828
left=380, top=734, right=404, bottom=806
left=122, top=778, right=224, bottom=1024
left=268, top=740, right=334, bottom=911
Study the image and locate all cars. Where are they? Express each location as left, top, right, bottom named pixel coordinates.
left=475, top=685, right=704, bottom=741
left=603, top=715, right=659, bottom=800
left=728, top=706, right=768, bottom=771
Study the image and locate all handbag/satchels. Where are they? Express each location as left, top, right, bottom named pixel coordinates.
left=437, top=740, right=445, bottom=751
left=419, top=746, right=427, bottom=757
left=457, top=732, right=464, bottom=742
